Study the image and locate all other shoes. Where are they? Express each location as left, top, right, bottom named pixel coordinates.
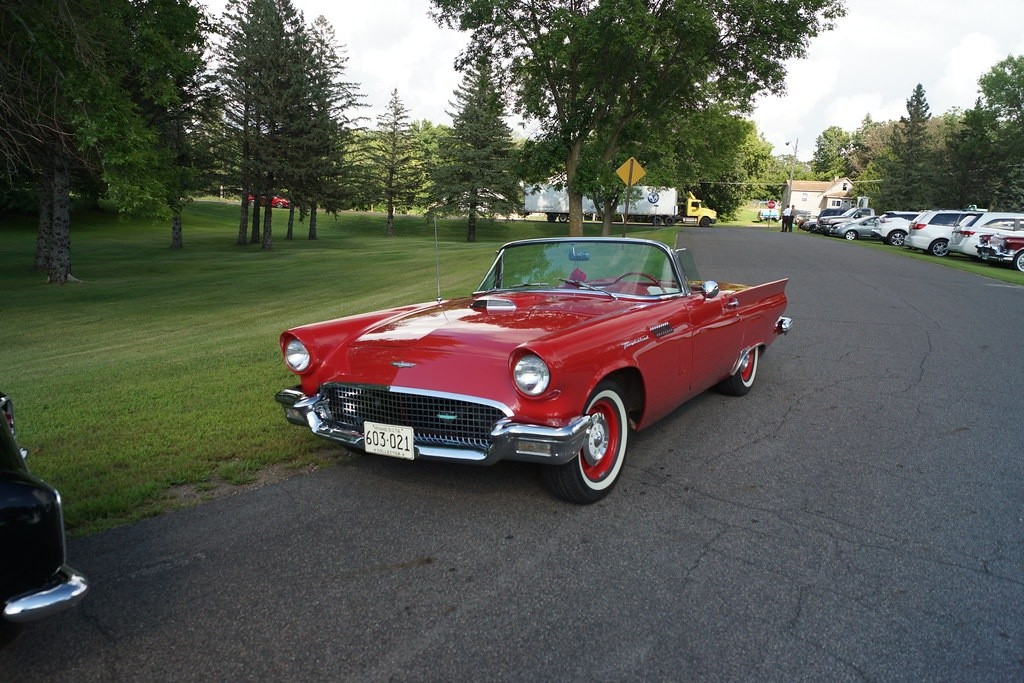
left=780, top=231, right=784, bottom=232
left=789, top=230, right=792, bottom=232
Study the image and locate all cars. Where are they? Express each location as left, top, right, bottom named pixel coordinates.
left=248, top=193, right=290, bottom=208
left=795, top=204, right=1024, bottom=271
left=757, top=207, right=779, bottom=223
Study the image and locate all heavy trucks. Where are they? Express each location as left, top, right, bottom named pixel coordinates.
left=523, top=184, right=718, bottom=227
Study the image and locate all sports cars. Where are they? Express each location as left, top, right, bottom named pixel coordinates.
left=274, top=236, right=794, bottom=507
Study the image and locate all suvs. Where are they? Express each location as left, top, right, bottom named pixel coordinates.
left=0, top=388, right=92, bottom=645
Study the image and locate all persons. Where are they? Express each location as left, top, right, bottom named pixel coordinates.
left=780, top=204, right=796, bottom=232
